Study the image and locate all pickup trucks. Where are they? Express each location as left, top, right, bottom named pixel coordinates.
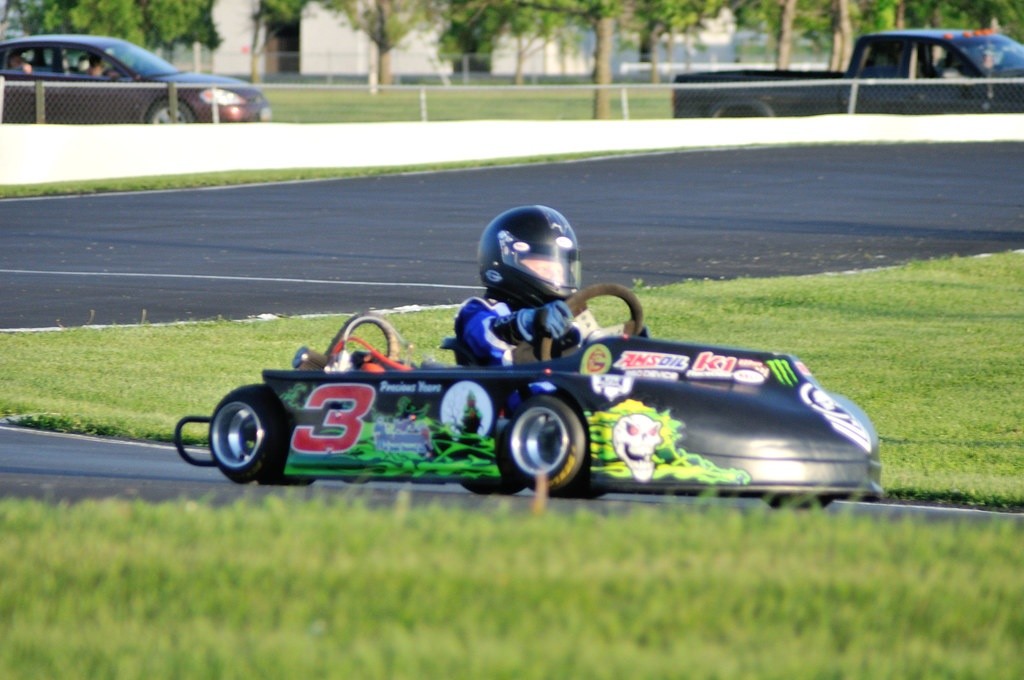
left=672, top=30, right=1024, bottom=119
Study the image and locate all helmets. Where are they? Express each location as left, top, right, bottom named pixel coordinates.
left=478, top=205, right=581, bottom=309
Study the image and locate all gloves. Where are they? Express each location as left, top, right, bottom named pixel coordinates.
left=639, top=326, right=648, bottom=337
left=516, top=300, right=575, bottom=342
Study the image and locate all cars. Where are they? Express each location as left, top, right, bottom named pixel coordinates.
left=1, top=35, right=275, bottom=124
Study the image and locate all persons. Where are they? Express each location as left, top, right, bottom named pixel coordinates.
left=82, top=54, right=104, bottom=76
left=7, top=53, right=33, bottom=74
left=453, top=205, right=647, bottom=372
